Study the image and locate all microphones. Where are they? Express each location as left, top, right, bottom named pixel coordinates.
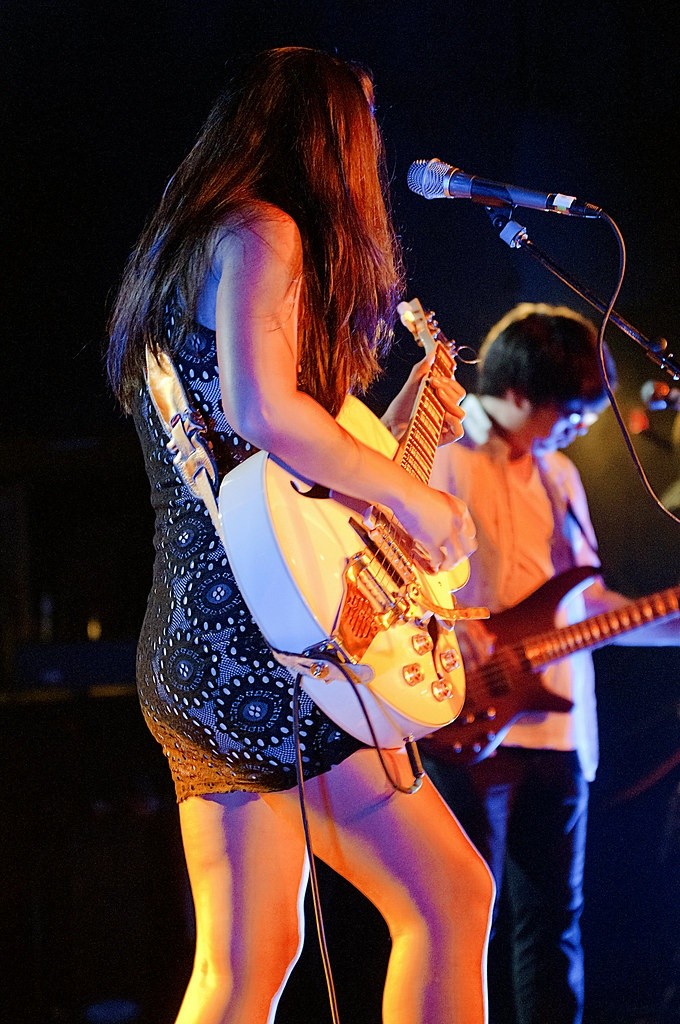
left=407, top=157, right=603, bottom=218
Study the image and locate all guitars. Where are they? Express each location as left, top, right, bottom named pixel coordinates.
left=417, top=565, right=680, bottom=773
left=219, top=297, right=473, bottom=748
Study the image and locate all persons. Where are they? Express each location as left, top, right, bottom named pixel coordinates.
left=105, top=47, right=495, bottom=1024
left=427, top=305, right=679, bottom=1024
